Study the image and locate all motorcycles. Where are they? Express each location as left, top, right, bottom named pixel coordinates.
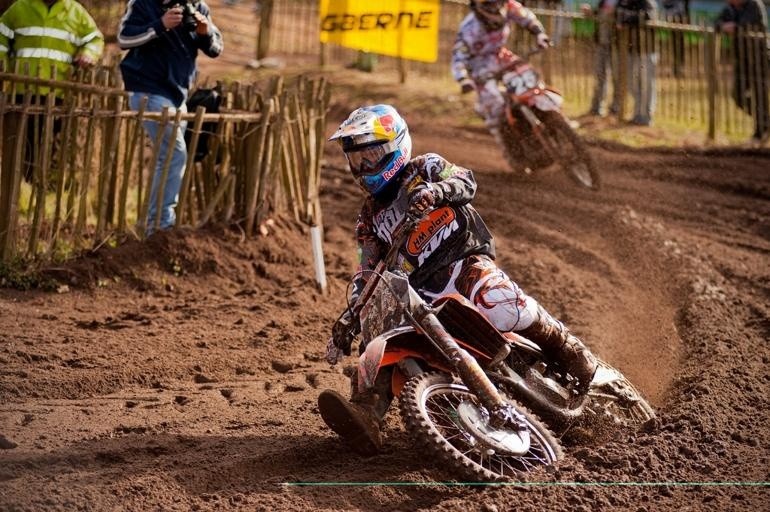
left=335, top=199, right=659, bottom=489
left=461, top=39, right=603, bottom=192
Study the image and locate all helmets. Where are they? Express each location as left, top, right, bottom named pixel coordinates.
left=469, top=0, right=509, bottom=29
left=327, top=102, right=413, bottom=196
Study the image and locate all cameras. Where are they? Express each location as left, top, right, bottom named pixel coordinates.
left=181, top=3, right=197, bottom=32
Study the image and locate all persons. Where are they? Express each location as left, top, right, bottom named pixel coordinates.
left=574, top=0, right=656, bottom=125
left=0, top=0, right=105, bottom=195
left=117, top=0, right=224, bottom=240
left=713, top=0, right=770, bottom=149
left=449, top=0, right=554, bottom=175
left=319, top=103, right=598, bottom=466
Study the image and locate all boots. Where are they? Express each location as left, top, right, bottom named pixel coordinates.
left=514, top=303, right=598, bottom=384
left=318, top=380, right=395, bottom=458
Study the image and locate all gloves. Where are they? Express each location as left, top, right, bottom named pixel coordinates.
left=406, top=186, right=435, bottom=225
left=537, top=32, right=550, bottom=50
left=461, top=78, right=477, bottom=91
left=331, top=310, right=361, bottom=350
